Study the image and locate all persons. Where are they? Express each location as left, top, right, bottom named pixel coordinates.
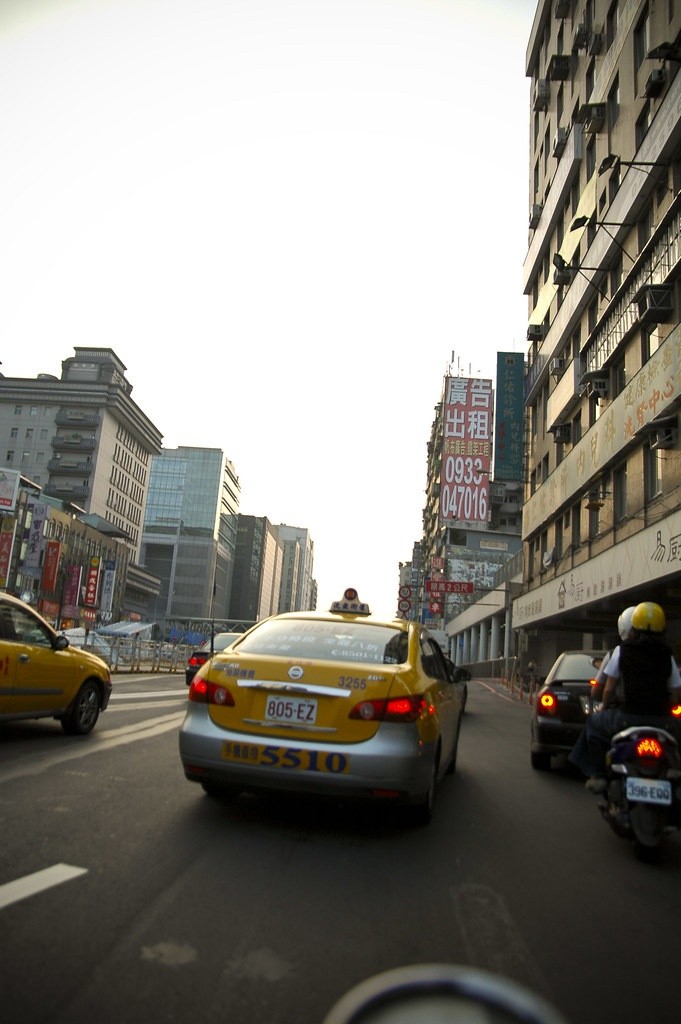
left=583, top=600, right=681, bottom=792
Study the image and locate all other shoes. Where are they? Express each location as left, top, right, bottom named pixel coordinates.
left=584, top=778, right=606, bottom=791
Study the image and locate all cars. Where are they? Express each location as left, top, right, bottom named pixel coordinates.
left=0, top=590, right=113, bottom=735
left=529, top=649, right=612, bottom=772
left=175, top=588, right=473, bottom=827
left=184, top=631, right=244, bottom=688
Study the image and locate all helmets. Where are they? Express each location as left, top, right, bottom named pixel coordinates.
left=618, top=606, right=636, bottom=640
left=630, top=602, right=664, bottom=631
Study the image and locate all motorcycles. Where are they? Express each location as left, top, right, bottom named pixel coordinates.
left=582, top=656, right=680, bottom=864
